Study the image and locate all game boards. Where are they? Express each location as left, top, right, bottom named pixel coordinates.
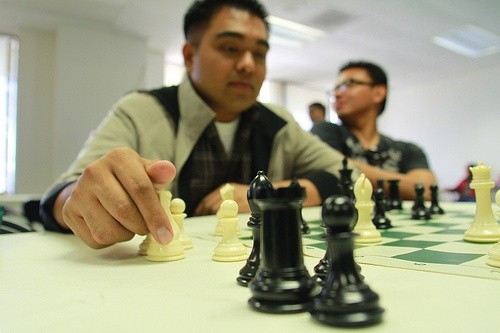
left=187, top=199, right=500, bottom=281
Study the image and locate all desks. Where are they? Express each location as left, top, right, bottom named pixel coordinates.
left=0, top=200, right=500, bottom=333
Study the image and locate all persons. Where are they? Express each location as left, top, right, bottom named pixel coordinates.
left=304, top=61, right=438, bottom=203
left=41, top=1, right=360, bottom=250
left=308, top=101, right=326, bottom=123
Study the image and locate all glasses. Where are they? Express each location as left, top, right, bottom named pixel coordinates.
left=326, top=79, right=376, bottom=97
